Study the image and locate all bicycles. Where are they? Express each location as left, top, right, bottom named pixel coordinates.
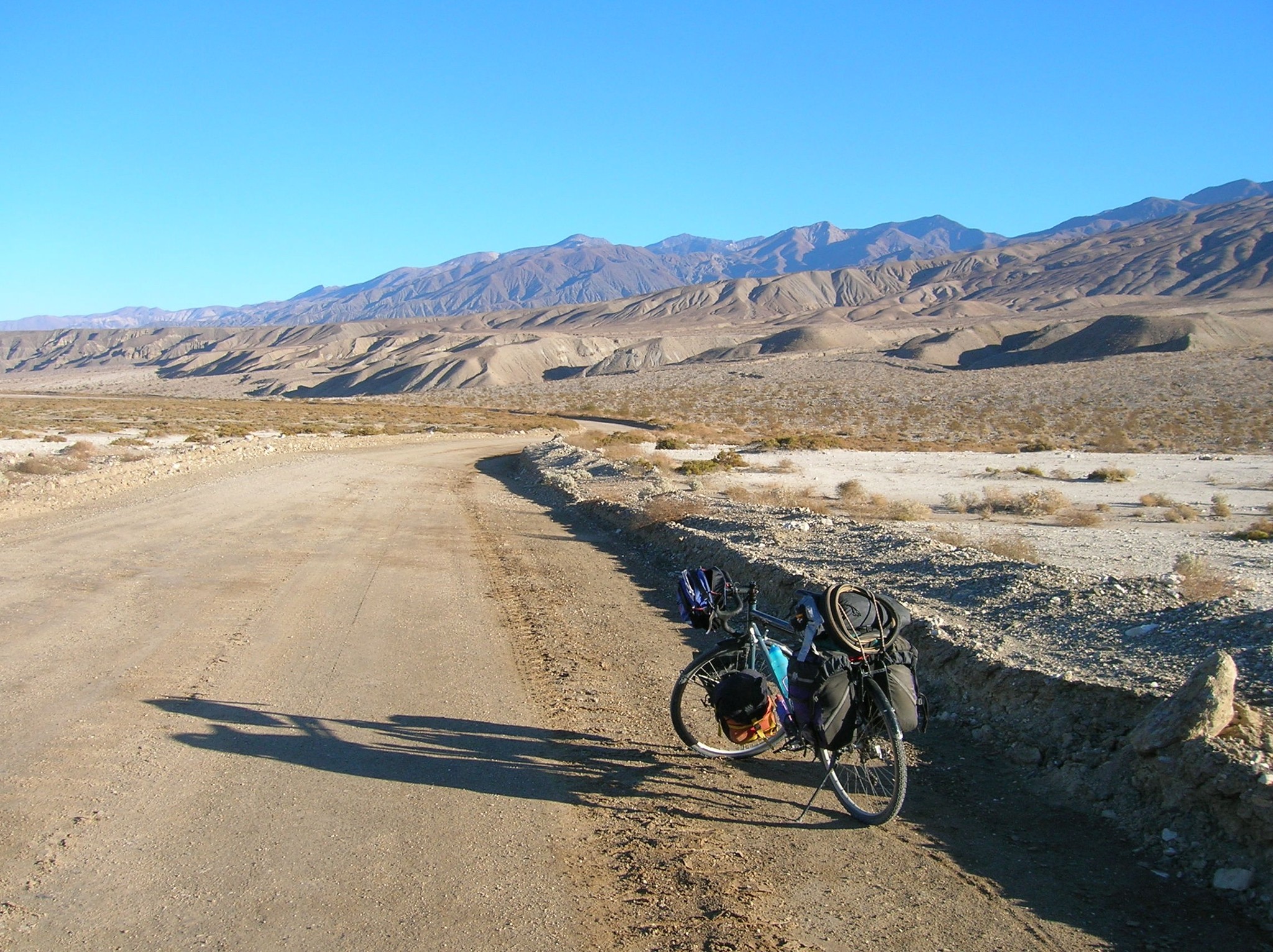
left=670, top=564, right=909, bottom=827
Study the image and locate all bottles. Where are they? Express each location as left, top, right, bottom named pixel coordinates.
left=775, top=694, right=797, bottom=736
left=768, top=643, right=790, bottom=698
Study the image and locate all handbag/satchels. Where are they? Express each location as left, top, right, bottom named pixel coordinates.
left=708, top=668, right=778, bottom=745
left=779, top=588, right=929, bottom=750
left=677, top=565, right=711, bottom=629
